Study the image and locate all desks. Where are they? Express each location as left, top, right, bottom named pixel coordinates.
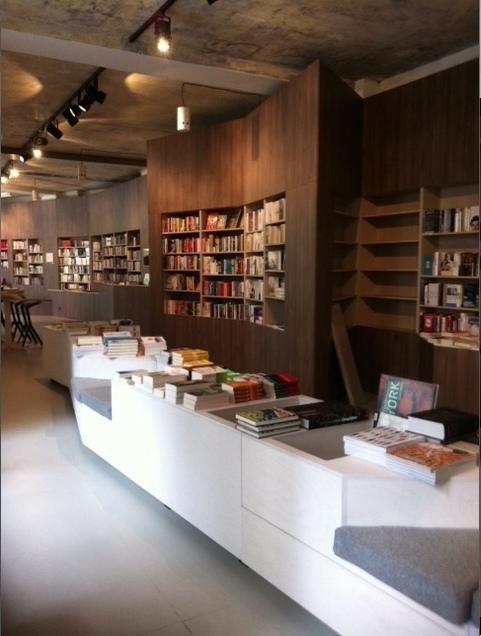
left=42, top=320, right=481, bottom=636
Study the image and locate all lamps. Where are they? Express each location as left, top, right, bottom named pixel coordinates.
left=153, top=15, right=173, bottom=53
left=1, top=66, right=107, bottom=184
left=176, top=83, right=191, bottom=133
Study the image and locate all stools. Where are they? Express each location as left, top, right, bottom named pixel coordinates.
left=0, top=299, right=44, bottom=347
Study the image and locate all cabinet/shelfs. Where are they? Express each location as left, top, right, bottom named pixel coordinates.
left=0, top=237, right=44, bottom=287
left=91, top=229, right=144, bottom=285
left=159, top=190, right=287, bottom=333
left=57, top=236, right=90, bottom=292
left=331, top=187, right=481, bottom=348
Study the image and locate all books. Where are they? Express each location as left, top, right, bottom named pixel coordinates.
left=234, top=400, right=369, bottom=439
left=160, top=196, right=288, bottom=316
left=421, top=202, right=481, bottom=315
left=53, top=316, right=167, bottom=359
left=116, top=347, right=300, bottom=411
left=2, top=237, right=43, bottom=288
left=57, top=231, right=149, bottom=294
left=343, top=374, right=481, bottom=486
